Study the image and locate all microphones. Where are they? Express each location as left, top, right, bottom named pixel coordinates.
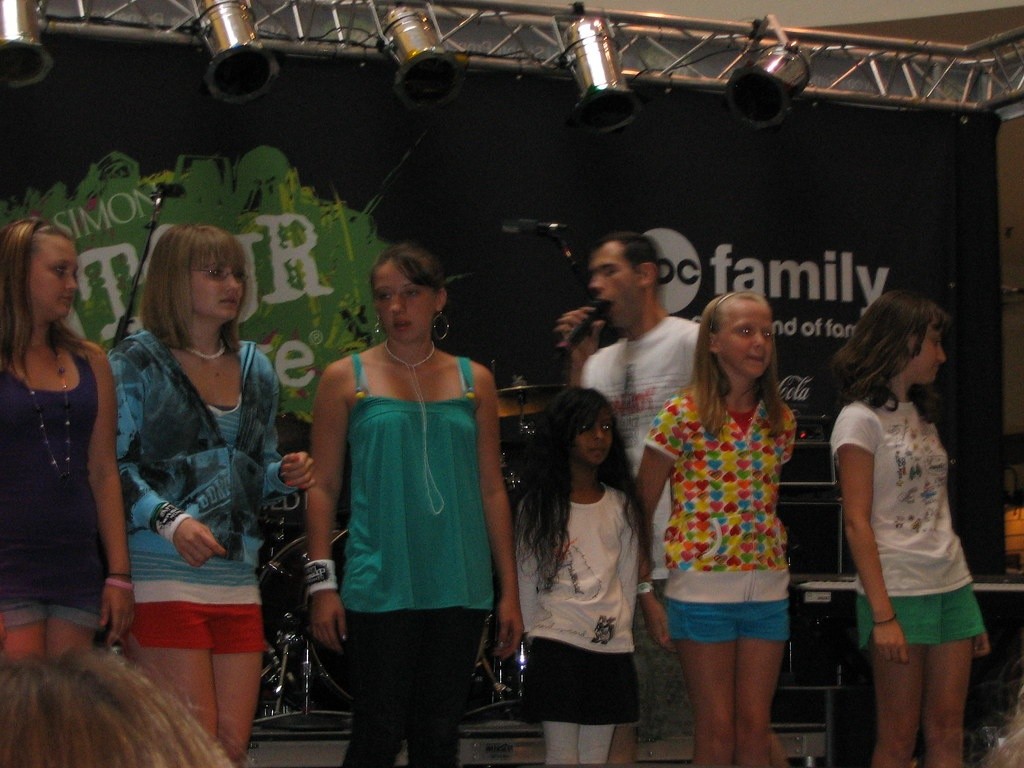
left=502, top=219, right=568, bottom=233
left=555, top=298, right=612, bottom=355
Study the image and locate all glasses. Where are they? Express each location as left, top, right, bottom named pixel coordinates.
left=188, top=266, right=249, bottom=284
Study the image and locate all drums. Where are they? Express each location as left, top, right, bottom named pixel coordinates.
left=303, top=528, right=491, bottom=705
left=254, top=519, right=309, bottom=604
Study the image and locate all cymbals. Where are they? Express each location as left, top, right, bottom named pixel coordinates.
left=494, top=381, right=569, bottom=418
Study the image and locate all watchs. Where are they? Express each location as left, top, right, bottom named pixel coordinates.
left=635, top=581, right=655, bottom=596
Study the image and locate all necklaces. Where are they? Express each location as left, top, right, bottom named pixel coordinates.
left=182, top=339, right=226, bottom=360
left=21, top=346, right=71, bottom=484
left=384, top=338, right=445, bottom=515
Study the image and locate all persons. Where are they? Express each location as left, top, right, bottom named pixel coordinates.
left=515, top=387, right=649, bottom=765
left=828, top=290, right=992, bottom=768
left=635, top=290, right=799, bottom=768
left=107, top=222, right=318, bottom=768
left=0, top=214, right=138, bottom=661
left=553, top=231, right=790, bottom=767
left=0, top=643, right=236, bottom=768
left=305, top=243, right=525, bottom=768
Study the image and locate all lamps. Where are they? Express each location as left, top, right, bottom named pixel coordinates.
left=0, top=0, right=811, bottom=139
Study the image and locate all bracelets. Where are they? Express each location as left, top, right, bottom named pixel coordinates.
left=104, top=571, right=134, bottom=593
left=872, top=612, right=897, bottom=627
left=305, top=559, right=337, bottom=595
